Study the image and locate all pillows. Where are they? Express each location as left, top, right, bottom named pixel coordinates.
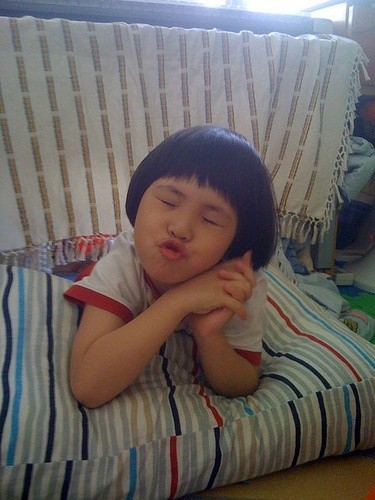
left=0, top=257, right=374, bottom=500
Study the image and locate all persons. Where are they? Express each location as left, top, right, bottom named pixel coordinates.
left=62, top=124, right=280, bottom=409
